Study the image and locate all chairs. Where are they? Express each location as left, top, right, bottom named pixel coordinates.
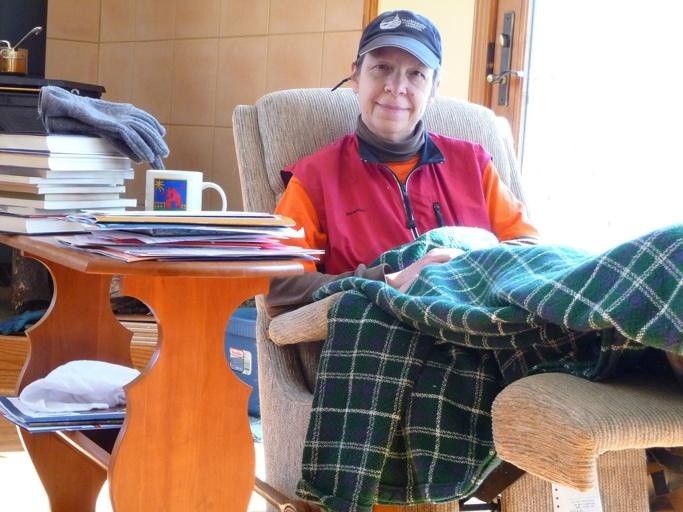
left=229, top=84, right=557, bottom=511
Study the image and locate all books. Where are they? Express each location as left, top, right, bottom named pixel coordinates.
left=0, top=394, right=126, bottom=435
left=0, top=132, right=137, bottom=236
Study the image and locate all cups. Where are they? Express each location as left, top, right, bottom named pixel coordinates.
left=145, top=169, right=227, bottom=212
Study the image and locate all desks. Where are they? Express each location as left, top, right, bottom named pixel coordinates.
left=1, top=206, right=308, bottom=510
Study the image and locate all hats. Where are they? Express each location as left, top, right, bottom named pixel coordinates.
left=357, top=9, right=442, bottom=70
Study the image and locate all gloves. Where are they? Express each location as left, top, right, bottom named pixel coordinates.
left=38, top=84, right=171, bottom=169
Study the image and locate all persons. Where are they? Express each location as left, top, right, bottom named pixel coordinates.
left=261, top=8, right=681, bottom=395
